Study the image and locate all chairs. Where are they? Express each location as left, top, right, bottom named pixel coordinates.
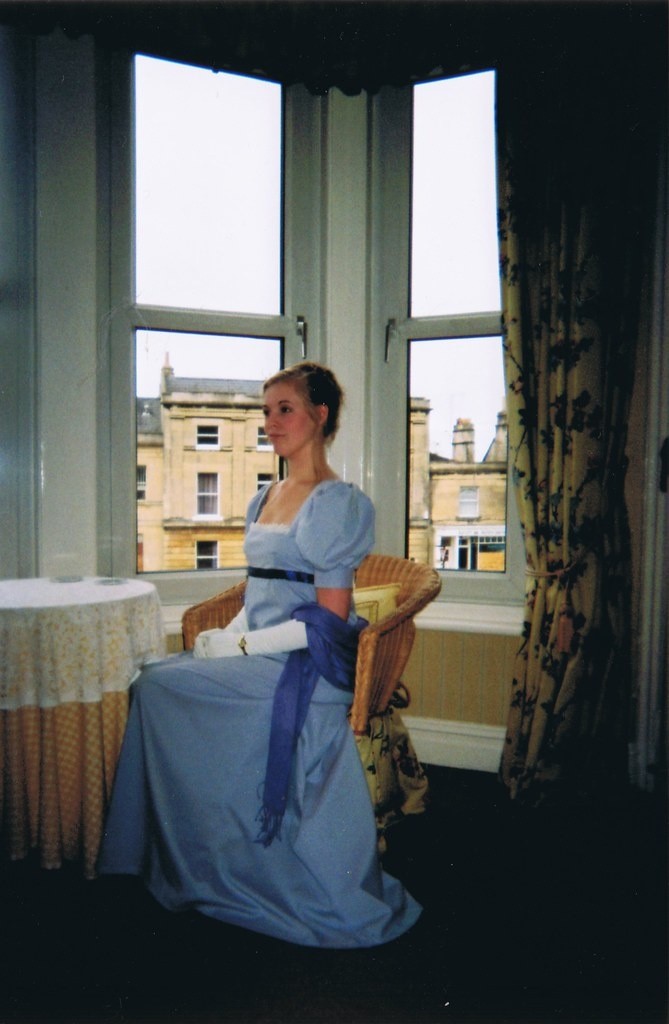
left=182, top=555, right=442, bottom=874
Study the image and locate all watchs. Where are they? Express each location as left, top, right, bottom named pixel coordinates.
left=239, top=633, right=248, bottom=655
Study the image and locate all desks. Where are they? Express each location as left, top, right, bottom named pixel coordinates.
left=0, top=574, right=167, bottom=881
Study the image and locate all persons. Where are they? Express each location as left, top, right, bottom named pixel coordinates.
left=95, top=361, right=424, bottom=948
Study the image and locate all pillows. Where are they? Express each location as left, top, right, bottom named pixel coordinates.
left=352, top=582, right=402, bottom=625
